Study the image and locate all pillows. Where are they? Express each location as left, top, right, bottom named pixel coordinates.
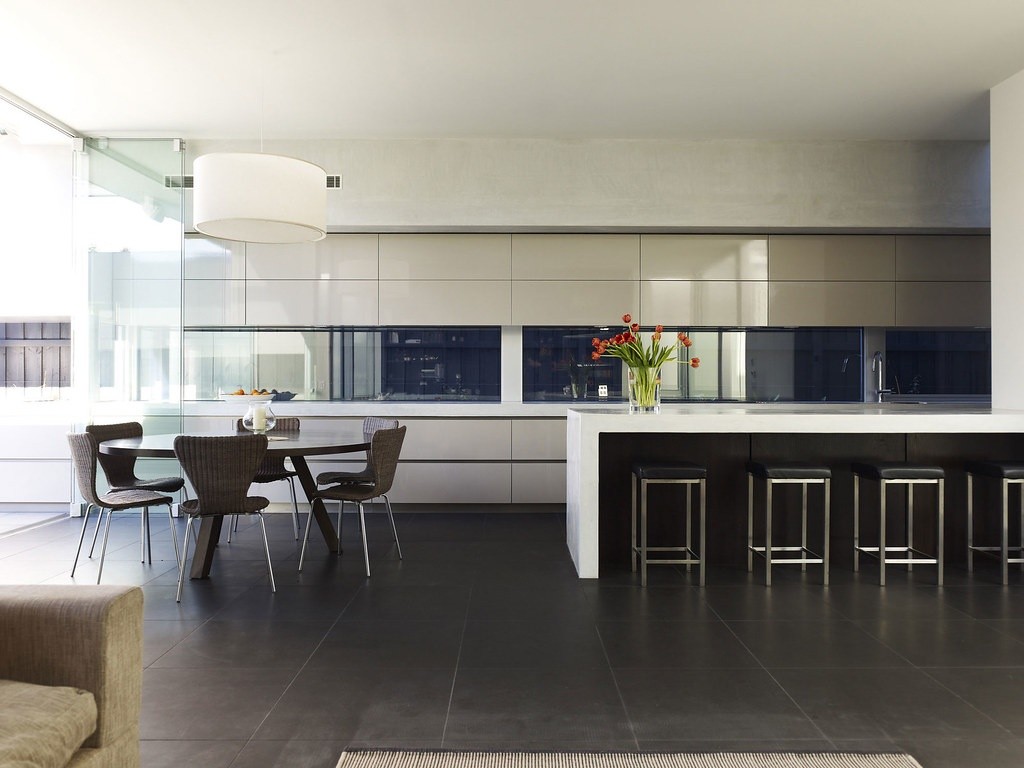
left=0, top=680, right=99, bottom=768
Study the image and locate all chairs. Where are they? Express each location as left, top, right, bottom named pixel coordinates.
left=317, top=417, right=398, bottom=557
left=298, top=425, right=403, bottom=576
left=66, top=430, right=180, bottom=584
left=85, top=421, right=198, bottom=566
left=228, top=416, right=300, bottom=542
left=174, top=434, right=276, bottom=603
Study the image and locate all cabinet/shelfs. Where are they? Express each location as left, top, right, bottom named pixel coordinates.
left=183, top=233, right=991, bottom=327
left=182, top=416, right=566, bottom=505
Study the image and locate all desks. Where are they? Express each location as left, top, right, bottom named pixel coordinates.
left=99, top=431, right=371, bottom=580
left=566, top=408, right=1024, bottom=579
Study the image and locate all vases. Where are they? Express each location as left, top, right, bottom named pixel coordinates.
left=627, top=366, right=662, bottom=416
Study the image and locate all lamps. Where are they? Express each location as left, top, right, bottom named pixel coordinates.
left=192, top=137, right=328, bottom=244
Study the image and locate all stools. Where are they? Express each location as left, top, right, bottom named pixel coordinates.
left=962, top=462, right=1023, bottom=587
left=628, top=461, right=707, bottom=586
left=852, top=464, right=945, bottom=588
left=745, top=460, right=832, bottom=586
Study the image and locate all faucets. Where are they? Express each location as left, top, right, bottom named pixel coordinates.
left=872, top=351, right=891, bottom=403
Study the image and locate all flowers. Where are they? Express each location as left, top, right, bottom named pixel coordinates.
left=591, top=314, right=699, bottom=369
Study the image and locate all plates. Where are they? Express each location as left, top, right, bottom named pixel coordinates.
left=220, top=393, right=275, bottom=400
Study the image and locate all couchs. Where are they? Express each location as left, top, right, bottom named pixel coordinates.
left=0, top=584, right=144, bottom=768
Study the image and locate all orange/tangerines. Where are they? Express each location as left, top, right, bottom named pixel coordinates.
left=231, top=389, right=268, bottom=395
